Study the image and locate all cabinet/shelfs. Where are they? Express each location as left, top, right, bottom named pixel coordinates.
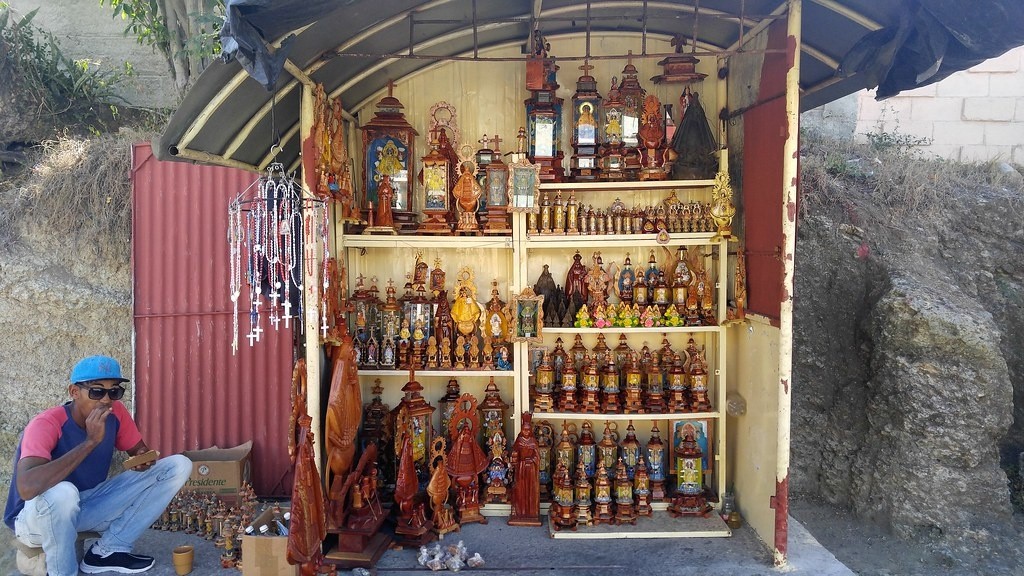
left=516, top=153, right=733, bottom=519
left=337, top=216, right=516, bottom=514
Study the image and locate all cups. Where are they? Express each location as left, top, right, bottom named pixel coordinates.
left=172, top=545, right=194, bottom=576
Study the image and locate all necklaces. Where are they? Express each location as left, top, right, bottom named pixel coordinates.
left=227, top=171, right=329, bottom=356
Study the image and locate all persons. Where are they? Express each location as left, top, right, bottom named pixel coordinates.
left=376, top=173, right=393, bottom=227
left=680, top=85, right=693, bottom=118
left=510, top=412, right=541, bottom=520
left=3, top=354, right=193, bottom=576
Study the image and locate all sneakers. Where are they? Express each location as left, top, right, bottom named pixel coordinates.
left=79, top=541, right=156, bottom=573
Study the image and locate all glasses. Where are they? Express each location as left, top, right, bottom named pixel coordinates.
left=76, top=383, right=125, bottom=401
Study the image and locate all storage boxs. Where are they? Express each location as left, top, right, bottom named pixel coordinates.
left=122, top=449, right=161, bottom=470
left=176, top=439, right=254, bottom=512
left=236, top=506, right=298, bottom=576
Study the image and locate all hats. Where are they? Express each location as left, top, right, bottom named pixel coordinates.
left=71, top=356, right=130, bottom=385
